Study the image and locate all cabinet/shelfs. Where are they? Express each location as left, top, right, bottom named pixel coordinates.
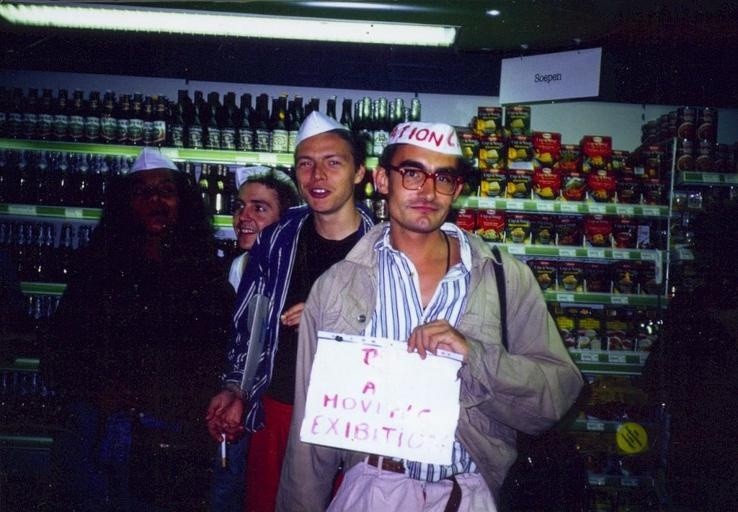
left=0, top=136, right=736, bottom=509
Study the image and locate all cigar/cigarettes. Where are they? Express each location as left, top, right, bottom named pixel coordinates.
left=220, top=431, right=228, bottom=468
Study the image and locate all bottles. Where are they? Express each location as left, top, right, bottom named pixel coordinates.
left=0, top=291, right=69, bottom=436
left=0, top=81, right=425, bottom=283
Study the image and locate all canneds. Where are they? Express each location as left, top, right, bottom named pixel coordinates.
left=450, top=104, right=669, bottom=205
left=514, top=255, right=658, bottom=296
left=546, top=300, right=662, bottom=349
left=641, top=106, right=736, bottom=174
left=447, top=209, right=667, bottom=252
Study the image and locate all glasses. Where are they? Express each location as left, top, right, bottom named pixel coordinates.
left=390, top=164, right=464, bottom=195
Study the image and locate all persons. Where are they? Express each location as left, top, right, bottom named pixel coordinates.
left=637, top=193, right=738, bottom=511
left=35, top=147, right=236, bottom=512
left=197, top=168, right=300, bottom=512
left=273, top=121, right=585, bottom=512
left=203, top=111, right=381, bottom=512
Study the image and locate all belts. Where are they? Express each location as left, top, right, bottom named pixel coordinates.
left=362, top=454, right=461, bottom=512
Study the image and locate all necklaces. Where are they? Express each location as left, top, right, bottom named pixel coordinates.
left=422, top=228, right=452, bottom=312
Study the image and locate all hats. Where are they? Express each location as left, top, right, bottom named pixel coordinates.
left=295, top=110, right=351, bottom=145
left=387, top=121, right=463, bottom=156
left=235, top=166, right=299, bottom=196
left=126, top=148, right=180, bottom=175
